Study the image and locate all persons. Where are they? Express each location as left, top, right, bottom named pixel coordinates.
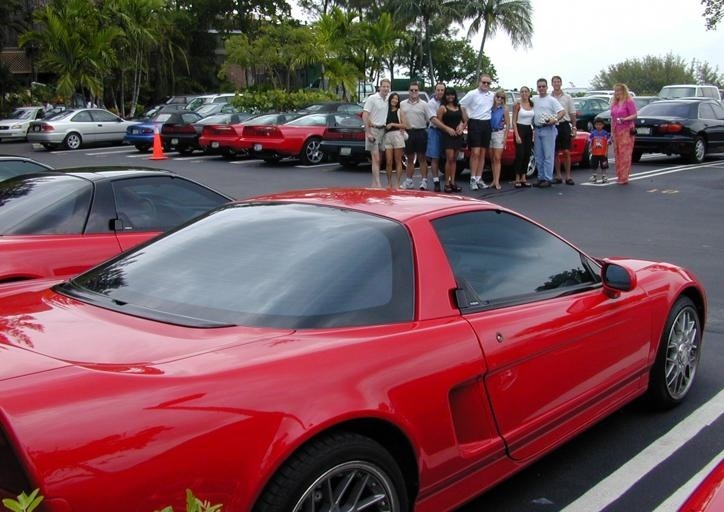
left=610, top=83, right=638, bottom=184
left=362, top=73, right=578, bottom=193
left=42, top=100, right=52, bottom=112
left=88, top=100, right=97, bottom=110
left=589, top=117, right=611, bottom=184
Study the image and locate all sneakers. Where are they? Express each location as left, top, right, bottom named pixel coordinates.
left=588, top=174, right=597, bottom=183
left=516, top=178, right=574, bottom=188
left=470, top=174, right=502, bottom=191
left=601, top=175, right=608, bottom=183
left=400, top=178, right=462, bottom=192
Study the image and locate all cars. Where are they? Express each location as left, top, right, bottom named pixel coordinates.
left=319, top=109, right=432, bottom=172
left=1, top=157, right=57, bottom=181
left=26, top=108, right=145, bottom=150
left=676, top=458, right=724, bottom=512
left=629, top=97, right=724, bottom=162
left=438, top=112, right=598, bottom=179
left=141, top=94, right=247, bottom=121
left=0, top=107, right=62, bottom=142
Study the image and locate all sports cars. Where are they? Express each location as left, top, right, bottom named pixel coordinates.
left=240, top=111, right=361, bottom=166
left=161, top=112, right=256, bottom=158
left=0, top=166, right=247, bottom=291
left=122, top=111, right=204, bottom=153
left=0, top=190, right=708, bottom=512
left=199, top=112, right=306, bottom=160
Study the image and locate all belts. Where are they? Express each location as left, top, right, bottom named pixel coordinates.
left=492, top=129, right=503, bottom=132
left=535, top=125, right=548, bottom=128
left=373, top=126, right=383, bottom=129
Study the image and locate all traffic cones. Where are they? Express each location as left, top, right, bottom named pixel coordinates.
left=147, top=126, right=169, bottom=160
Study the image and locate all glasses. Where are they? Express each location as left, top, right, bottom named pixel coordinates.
left=483, top=81, right=490, bottom=85
left=410, top=89, right=418, bottom=92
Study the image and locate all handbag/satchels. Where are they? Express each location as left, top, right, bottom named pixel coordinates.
left=403, top=131, right=409, bottom=141
left=630, top=127, right=637, bottom=135
